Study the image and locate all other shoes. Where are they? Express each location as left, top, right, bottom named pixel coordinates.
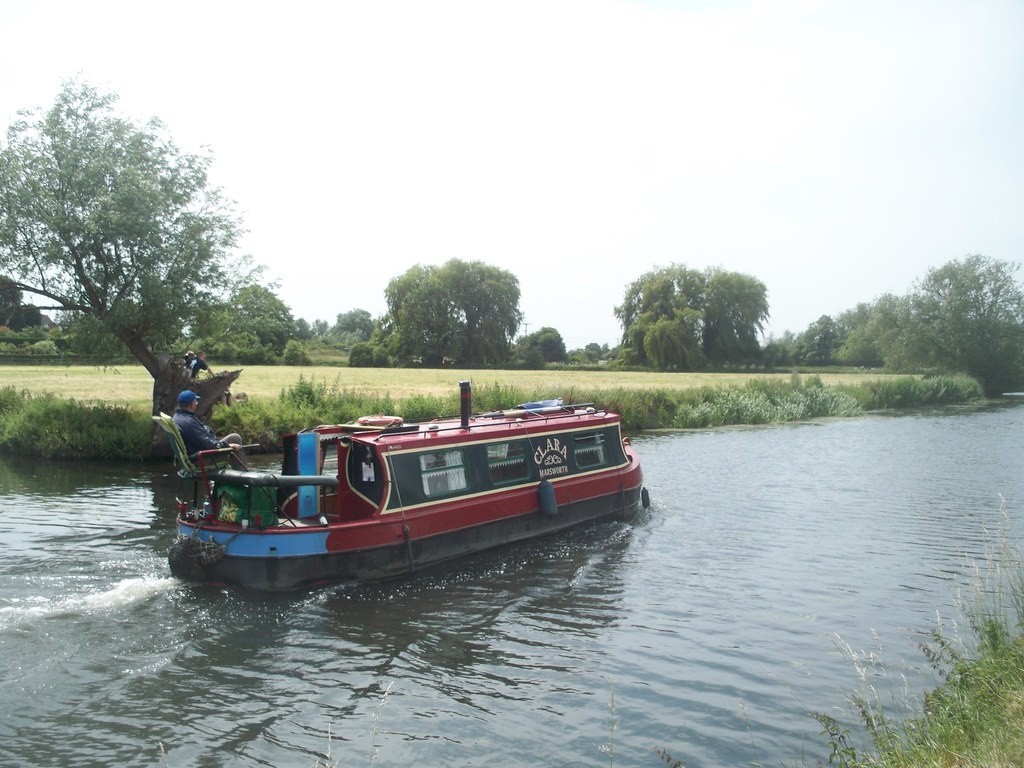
left=248, top=468, right=257, bottom=473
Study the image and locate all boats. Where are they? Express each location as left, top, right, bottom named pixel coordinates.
left=153, top=379, right=647, bottom=597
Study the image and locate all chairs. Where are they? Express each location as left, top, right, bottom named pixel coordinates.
left=152, top=411, right=231, bottom=518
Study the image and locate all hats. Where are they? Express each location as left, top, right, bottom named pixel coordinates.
left=178, top=390, right=200, bottom=404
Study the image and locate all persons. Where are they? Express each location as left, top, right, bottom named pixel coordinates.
left=184, top=350, right=215, bottom=381
left=171, top=390, right=249, bottom=471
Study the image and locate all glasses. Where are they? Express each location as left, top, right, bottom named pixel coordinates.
left=192, top=401, right=199, bottom=405
